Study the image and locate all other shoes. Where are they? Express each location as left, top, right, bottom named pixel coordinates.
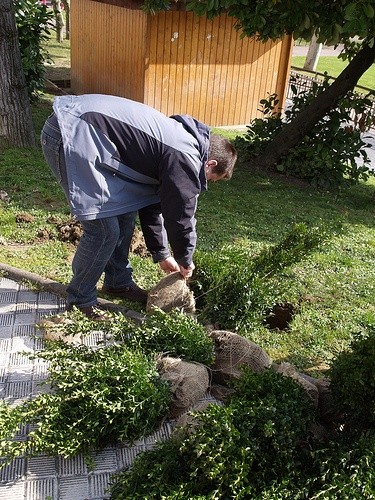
left=67, top=303, right=115, bottom=321
left=102, top=282, right=149, bottom=301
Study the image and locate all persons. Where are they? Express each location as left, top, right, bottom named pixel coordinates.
left=40, top=93, right=237, bottom=319
